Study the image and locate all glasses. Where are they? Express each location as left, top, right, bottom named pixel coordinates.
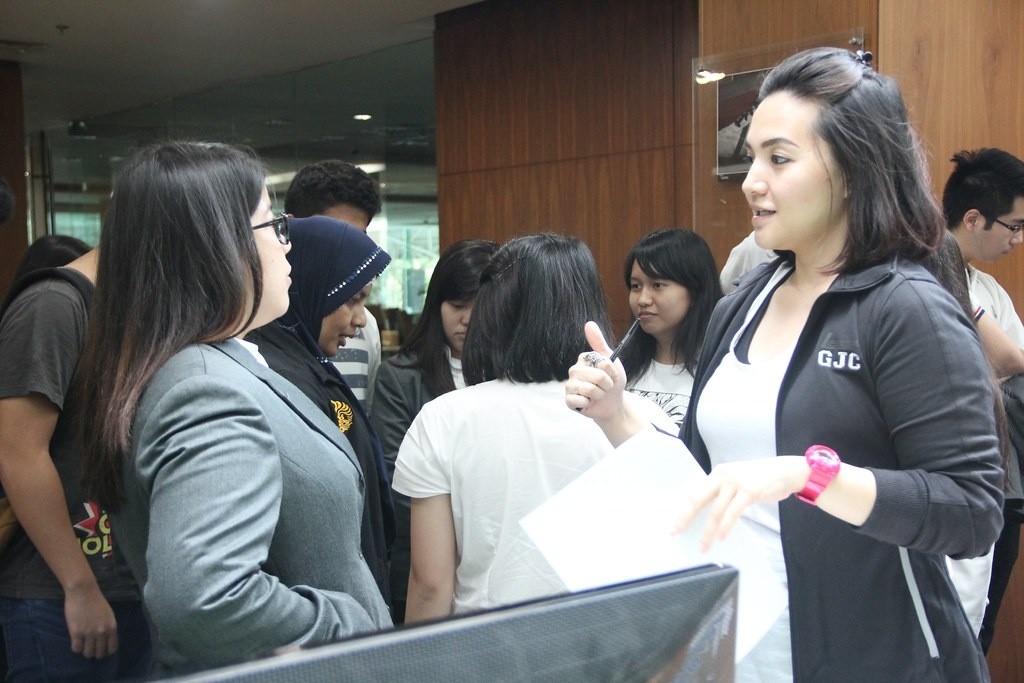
left=252, top=212, right=290, bottom=245
left=994, top=218, right=1024, bottom=235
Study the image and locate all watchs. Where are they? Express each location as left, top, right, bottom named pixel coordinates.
left=795, top=445, right=841, bottom=506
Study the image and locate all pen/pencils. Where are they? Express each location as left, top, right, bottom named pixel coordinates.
left=574, top=317, right=642, bottom=413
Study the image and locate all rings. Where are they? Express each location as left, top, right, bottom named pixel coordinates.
left=583, top=353, right=605, bottom=368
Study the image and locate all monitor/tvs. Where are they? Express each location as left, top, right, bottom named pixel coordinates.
left=158, top=567, right=742, bottom=683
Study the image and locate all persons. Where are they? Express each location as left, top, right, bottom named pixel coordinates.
left=720, top=231, right=779, bottom=295
left=917, top=148, right=1024, bottom=656
left=566, top=47, right=1010, bottom=683
left=1, top=140, right=725, bottom=683
